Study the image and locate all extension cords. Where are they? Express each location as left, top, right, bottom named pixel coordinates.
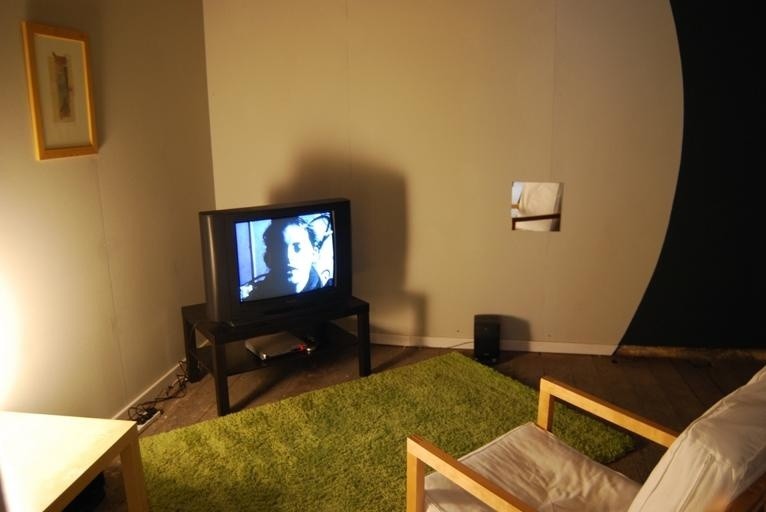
left=136, top=409, right=161, bottom=436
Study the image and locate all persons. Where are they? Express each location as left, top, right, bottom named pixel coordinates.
left=235, top=215, right=332, bottom=303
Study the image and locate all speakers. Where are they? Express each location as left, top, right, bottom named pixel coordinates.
left=474, top=315, right=500, bottom=366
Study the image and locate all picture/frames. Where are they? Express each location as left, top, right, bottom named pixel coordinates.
left=21, top=20, right=97, bottom=161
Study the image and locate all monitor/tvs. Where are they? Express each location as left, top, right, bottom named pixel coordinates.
left=199, top=197, right=352, bottom=328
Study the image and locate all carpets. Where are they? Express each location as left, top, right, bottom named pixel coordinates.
left=124, top=347, right=639, bottom=512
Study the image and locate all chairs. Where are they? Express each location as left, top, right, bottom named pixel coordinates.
left=403, top=365, right=766, bottom=512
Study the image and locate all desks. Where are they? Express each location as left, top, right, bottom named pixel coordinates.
left=182, top=296, right=371, bottom=417
left=1, top=411, right=152, bottom=512
